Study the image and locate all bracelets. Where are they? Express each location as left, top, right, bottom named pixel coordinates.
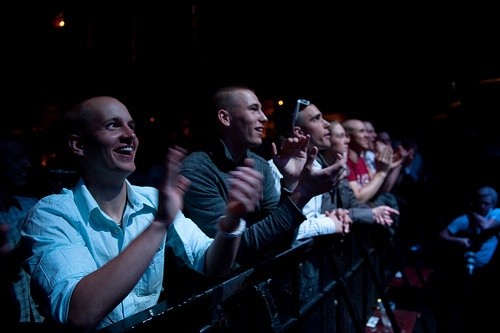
left=216, top=215, right=246, bottom=239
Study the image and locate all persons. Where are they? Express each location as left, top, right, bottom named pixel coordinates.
left=0, top=196, right=45, bottom=323
left=317, top=113, right=416, bottom=231
left=0, top=222, right=21, bottom=322
left=22, top=96, right=264, bottom=333
left=164, top=82, right=346, bottom=305
left=441, top=187, right=500, bottom=269
left=264, top=98, right=353, bottom=304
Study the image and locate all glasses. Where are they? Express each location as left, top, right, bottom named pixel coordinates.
left=293, top=98, right=310, bottom=126
